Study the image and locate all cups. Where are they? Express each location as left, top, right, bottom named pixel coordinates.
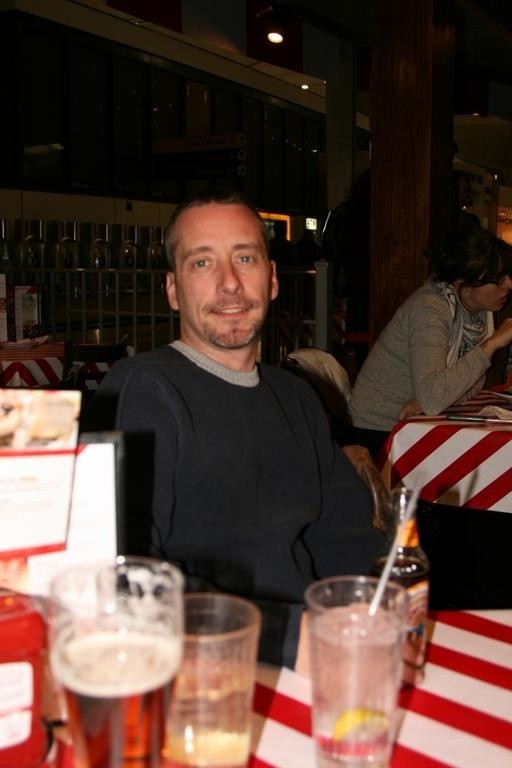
left=302, top=575, right=409, bottom=768
left=47, top=557, right=184, bottom=768
left=161, top=592, right=261, bottom=767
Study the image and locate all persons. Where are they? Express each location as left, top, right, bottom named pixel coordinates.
left=83, top=194, right=427, bottom=690
left=350, top=226, right=512, bottom=472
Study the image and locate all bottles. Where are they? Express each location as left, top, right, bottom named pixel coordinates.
left=377, top=489, right=432, bottom=683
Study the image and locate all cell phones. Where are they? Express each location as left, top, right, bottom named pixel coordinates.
left=447, top=414, right=487, bottom=421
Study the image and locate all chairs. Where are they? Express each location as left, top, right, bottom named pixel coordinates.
left=68, top=342, right=134, bottom=391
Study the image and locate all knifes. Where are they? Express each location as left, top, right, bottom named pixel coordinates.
left=447, top=412, right=512, bottom=426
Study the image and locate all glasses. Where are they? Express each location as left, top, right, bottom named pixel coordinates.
left=485, top=277, right=506, bottom=285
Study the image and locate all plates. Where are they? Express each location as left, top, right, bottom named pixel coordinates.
left=492, top=392, right=511, bottom=403
